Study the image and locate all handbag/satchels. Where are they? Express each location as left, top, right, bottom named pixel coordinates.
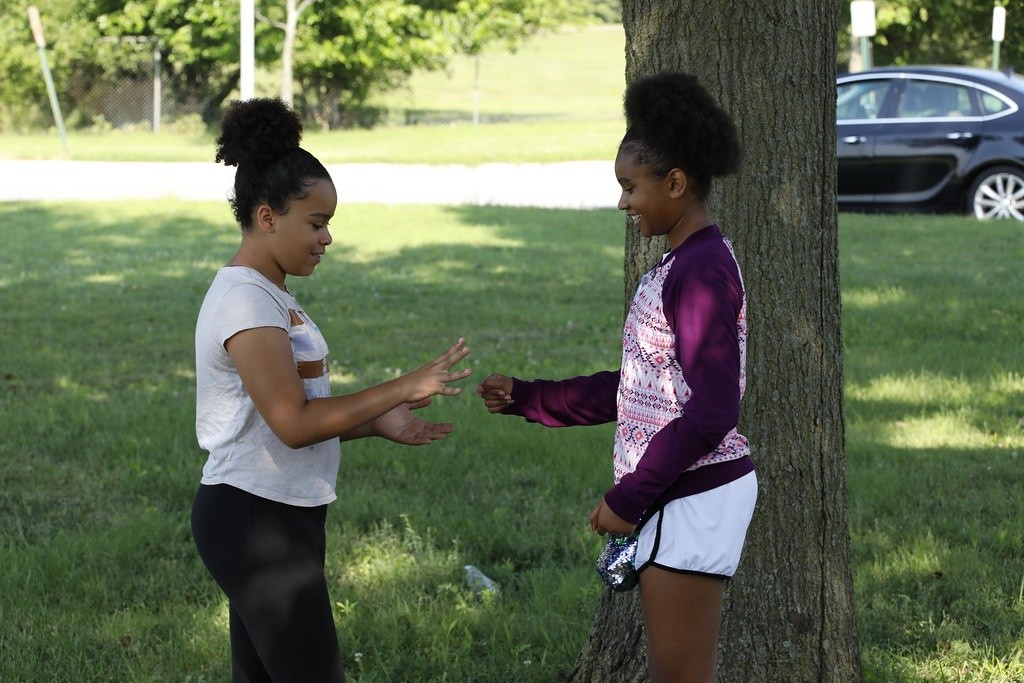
left=595, top=537, right=639, bottom=594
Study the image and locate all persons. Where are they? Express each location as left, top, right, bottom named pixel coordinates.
left=189, top=100, right=473, bottom=683
left=478, top=71, right=760, bottom=683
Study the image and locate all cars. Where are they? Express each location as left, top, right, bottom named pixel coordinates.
left=837, top=66, right=1023, bottom=221
